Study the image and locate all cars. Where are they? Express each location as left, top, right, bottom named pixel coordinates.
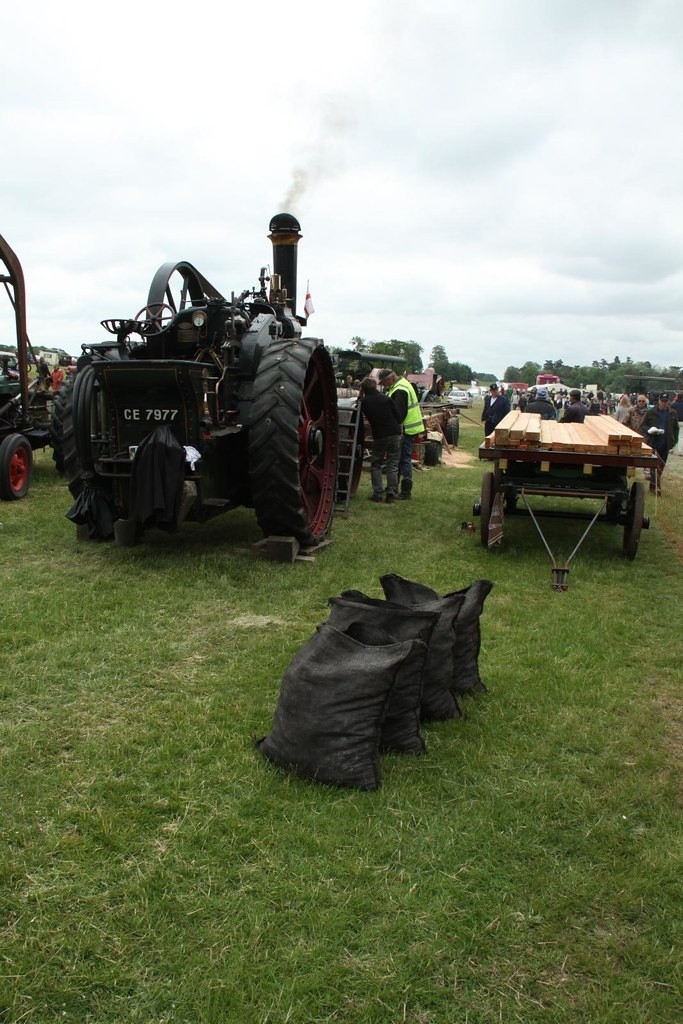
left=447, top=391, right=474, bottom=409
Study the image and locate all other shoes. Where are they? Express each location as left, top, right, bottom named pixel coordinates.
left=649, top=488, right=661, bottom=496
left=396, top=492, right=411, bottom=500
left=368, top=495, right=381, bottom=502
left=384, top=495, right=395, bottom=504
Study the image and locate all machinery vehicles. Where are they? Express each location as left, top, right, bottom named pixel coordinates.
left=66, top=213, right=363, bottom=554
left=0, top=234, right=75, bottom=500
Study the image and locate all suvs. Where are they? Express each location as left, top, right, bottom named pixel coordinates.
left=661, top=390, right=683, bottom=421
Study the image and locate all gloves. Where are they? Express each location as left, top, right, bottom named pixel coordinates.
left=648, top=426, right=665, bottom=435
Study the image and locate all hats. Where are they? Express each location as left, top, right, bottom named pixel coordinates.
left=660, top=392, right=669, bottom=401
left=377, top=370, right=393, bottom=384
left=489, top=383, right=499, bottom=391
left=569, top=390, right=581, bottom=396
left=535, top=387, right=548, bottom=398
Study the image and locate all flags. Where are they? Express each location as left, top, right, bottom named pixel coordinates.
left=303, top=286, right=314, bottom=319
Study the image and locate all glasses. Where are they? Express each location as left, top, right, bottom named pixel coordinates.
left=638, top=400, right=645, bottom=402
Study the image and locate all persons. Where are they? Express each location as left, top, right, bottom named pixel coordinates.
left=342, top=375, right=360, bottom=390
left=377, top=368, right=426, bottom=500
left=14, top=350, right=77, bottom=391
left=360, top=377, right=404, bottom=503
left=480, top=383, right=683, bottom=497
